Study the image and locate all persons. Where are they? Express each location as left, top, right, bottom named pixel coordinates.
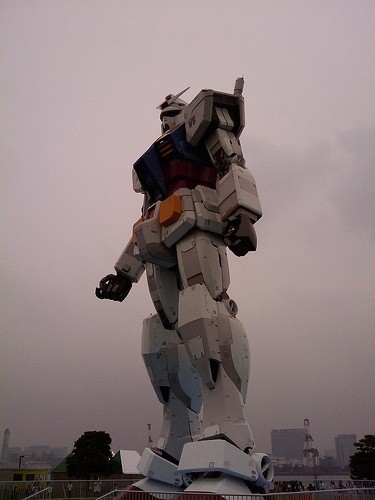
left=0, top=478, right=119, bottom=500
left=94, top=74, right=263, bottom=500
left=273, top=479, right=356, bottom=494
